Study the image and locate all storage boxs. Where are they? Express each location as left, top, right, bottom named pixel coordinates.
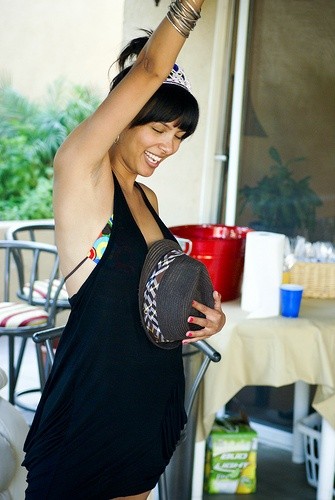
left=204, top=417, right=259, bottom=494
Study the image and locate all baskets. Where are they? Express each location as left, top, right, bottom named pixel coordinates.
left=289, top=263, right=335, bottom=298
left=297, top=413, right=335, bottom=496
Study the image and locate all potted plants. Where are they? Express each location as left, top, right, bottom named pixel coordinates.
left=237, top=147, right=323, bottom=235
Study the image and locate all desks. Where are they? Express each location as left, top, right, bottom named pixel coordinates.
left=191, top=295, right=335, bottom=500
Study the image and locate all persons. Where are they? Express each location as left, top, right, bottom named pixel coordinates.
left=20, top=0, right=225, bottom=500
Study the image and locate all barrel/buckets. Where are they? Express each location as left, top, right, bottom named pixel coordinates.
left=165, top=224, right=252, bottom=302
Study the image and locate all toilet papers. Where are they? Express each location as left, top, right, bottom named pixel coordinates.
left=239, top=229, right=296, bottom=316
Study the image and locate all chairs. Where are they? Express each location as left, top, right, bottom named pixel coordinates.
left=0, top=224, right=221, bottom=500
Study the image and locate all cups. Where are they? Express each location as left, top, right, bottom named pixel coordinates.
left=279, top=284, right=303, bottom=318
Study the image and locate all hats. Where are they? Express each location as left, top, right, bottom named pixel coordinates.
left=139, top=239, right=214, bottom=349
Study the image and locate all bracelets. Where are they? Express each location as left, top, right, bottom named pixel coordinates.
left=166, top=0, right=202, bottom=39
